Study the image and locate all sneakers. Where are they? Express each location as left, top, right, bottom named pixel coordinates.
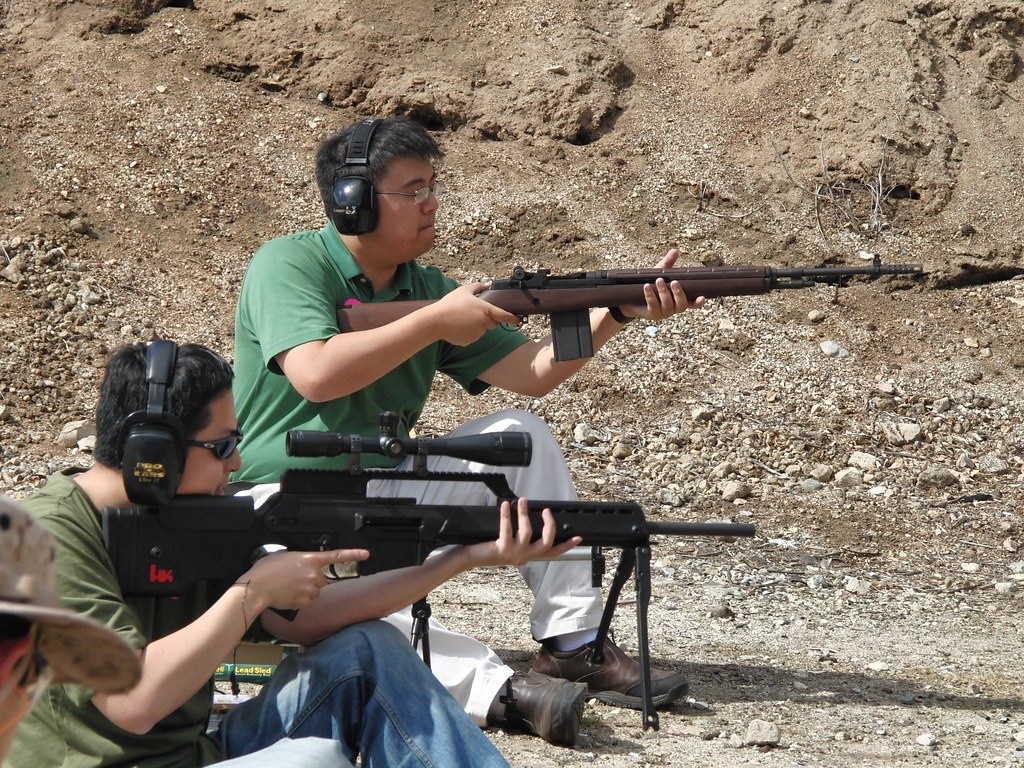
left=493, top=669, right=589, bottom=747
left=532, top=627, right=692, bottom=712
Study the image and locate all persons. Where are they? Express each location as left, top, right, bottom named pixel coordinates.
left=0, top=492, right=142, bottom=768
left=0, top=338, right=582, bottom=768
left=227, top=119, right=708, bottom=747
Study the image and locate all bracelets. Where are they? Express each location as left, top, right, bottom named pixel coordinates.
left=606, top=303, right=636, bottom=326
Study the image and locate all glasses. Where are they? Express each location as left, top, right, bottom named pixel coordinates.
left=186, top=423, right=246, bottom=460
left=374, top=180, right=447, bottom=204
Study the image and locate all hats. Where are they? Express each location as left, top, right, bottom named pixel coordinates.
left=0, top=496, right=145, bottom=696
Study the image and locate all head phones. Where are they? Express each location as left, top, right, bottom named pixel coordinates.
left=117, top=338, right=187, bottom=506
left=330, top=118, right=385, bottom=236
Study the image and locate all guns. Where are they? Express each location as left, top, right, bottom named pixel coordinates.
left=102, top=409, right=758, bottom=732
left=336, top=253, right=924, bottom=363
